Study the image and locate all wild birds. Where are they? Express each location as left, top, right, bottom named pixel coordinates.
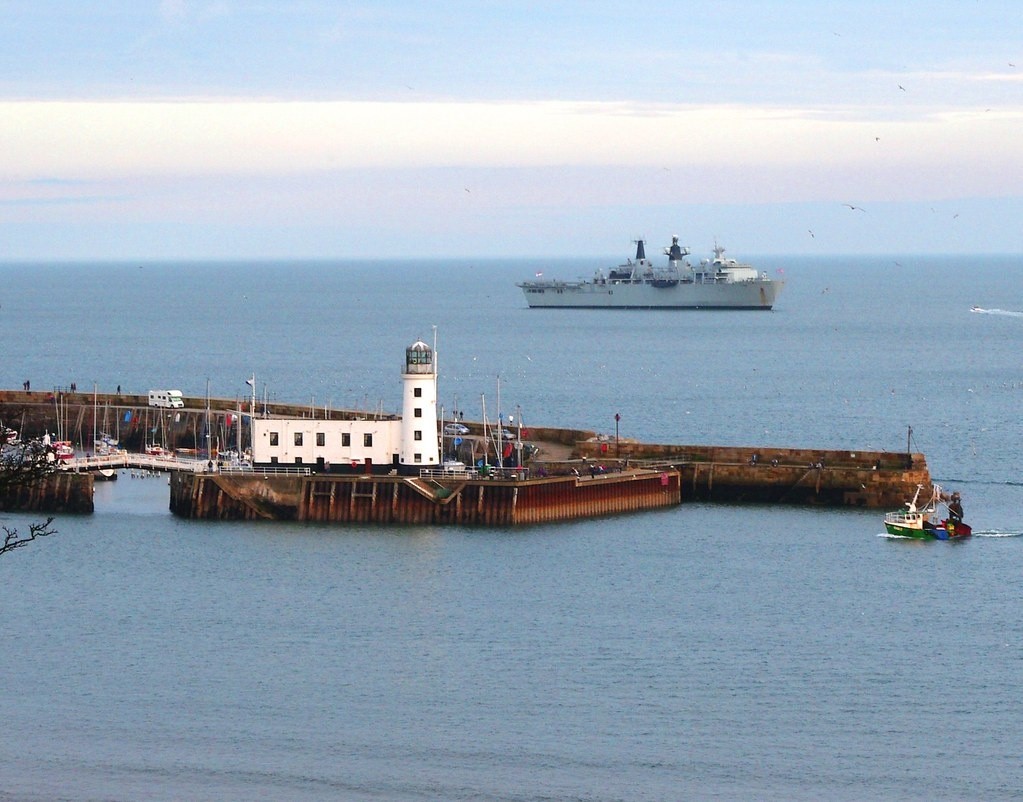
left=931, top=207, right=961, bottom=219
left=822, top=287, right=829, bottom=294
left=808, top=229, right=815, bottom=240
left=1007, top=62, right=1016, bottom=67
left=522, top=353, right=534, bottom=363
left=897, top=84, right=906, bottom=93
left=469, top=354, right=480, bottom=362
left=892, top=259, right=902, bottom=267
left=985, top=108, right=991, bottom=112
left=875, top=136, right=881, bottom=144
left=842, top=203, right=866, bottom=213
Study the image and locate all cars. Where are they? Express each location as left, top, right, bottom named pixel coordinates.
left=444, top=423, right=469, bottom=436
left=488, top=429, right=516, bottom=440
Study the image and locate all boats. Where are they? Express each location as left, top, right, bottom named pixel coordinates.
left=882, top=481, right=972, bottom=539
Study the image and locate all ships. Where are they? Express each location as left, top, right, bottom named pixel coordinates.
left=515, top=234, right=787, bottom=311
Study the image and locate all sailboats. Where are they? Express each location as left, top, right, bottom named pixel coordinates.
left=0, top=379, right=253, bottom=482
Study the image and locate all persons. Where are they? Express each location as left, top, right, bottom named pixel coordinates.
left=116, top=385, right=121, bottom=395
left=70, top=382, right=74, bottom=393
left=208, top=460, right=214, bottom=473
left=509, top=414, right=513, bottom=426
left=218, top=459, right=224, bottom=471
left=23, top=382, right=27, bottom=390
left=459, top=411, right=463, bottom=421
left=73, top=383, right=76, bottom=393
left=26, top=380, right=30, bottom=390
left=324, top=462, right=330, bottom=475
